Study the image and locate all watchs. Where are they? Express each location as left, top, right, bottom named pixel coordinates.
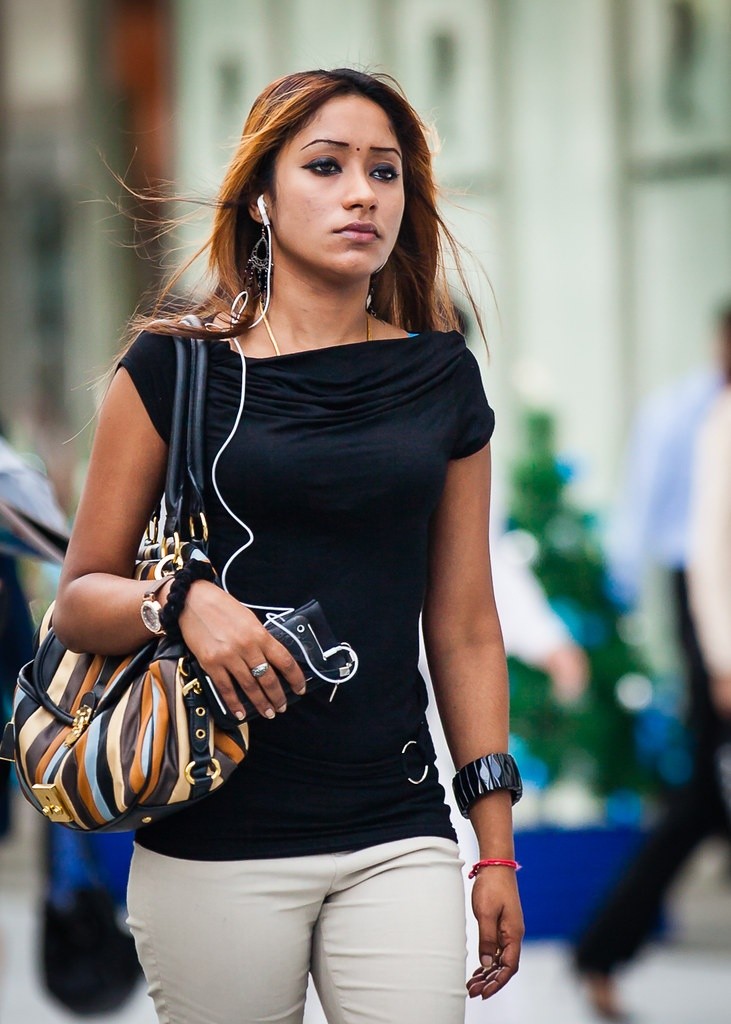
left=140, top=573, right=173, bottom=636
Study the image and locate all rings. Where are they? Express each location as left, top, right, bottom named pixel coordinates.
left=250, top=663, right=269, bottom=678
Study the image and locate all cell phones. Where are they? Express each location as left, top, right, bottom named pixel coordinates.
left=201, top=616, right=328, bottom=719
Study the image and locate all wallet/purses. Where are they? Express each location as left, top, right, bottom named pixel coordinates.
left=191, top=599, right=347, bottom=730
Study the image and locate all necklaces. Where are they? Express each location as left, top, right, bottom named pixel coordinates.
left=258, top=291, right=371, bottom=357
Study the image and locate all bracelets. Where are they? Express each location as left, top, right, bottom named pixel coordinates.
left=158, top=558, right=215, bottom=643
left=468, top=860, right=519, bottom=879
left=451, top=753, right=522, bottom=818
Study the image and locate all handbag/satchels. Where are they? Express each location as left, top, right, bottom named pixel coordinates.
left=0, top=315, right=250, bottom=833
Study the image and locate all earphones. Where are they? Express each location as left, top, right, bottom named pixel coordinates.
left=257, top=194, right=271, bottom=226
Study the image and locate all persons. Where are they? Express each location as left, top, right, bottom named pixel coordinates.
left=0, top=303, right=731, bottom=1024
left=50, top=65, right=523, bottom=1024
